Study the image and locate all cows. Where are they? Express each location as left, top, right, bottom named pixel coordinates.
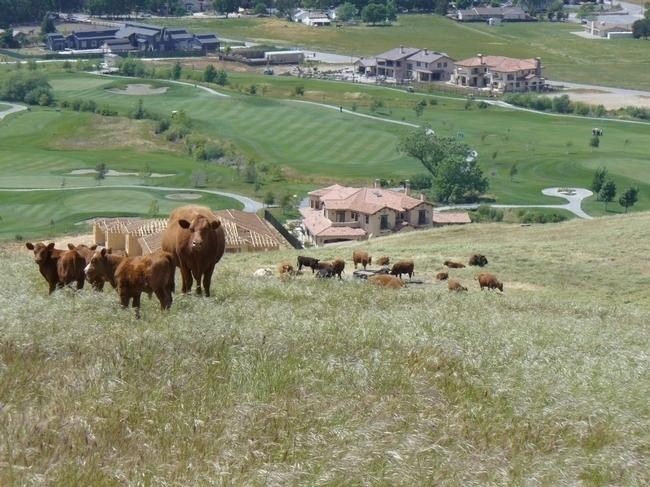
left=279, top=255, right=345, bottom=282
left=353, top=249, right=414, bottom=279
left=25, top=242, right=174, bottom=320
left=161, top=206, right=225, bottom=297
left=435, top=254, right=504, bottom=292
